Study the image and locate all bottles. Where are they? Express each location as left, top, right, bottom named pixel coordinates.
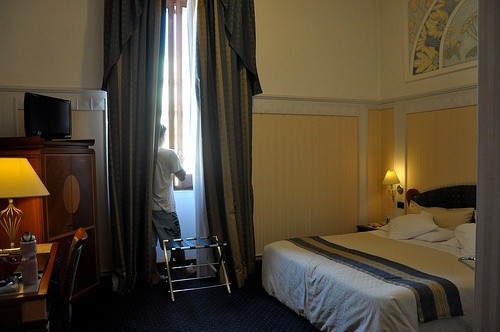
left=20, top=232, right=38, bottom=285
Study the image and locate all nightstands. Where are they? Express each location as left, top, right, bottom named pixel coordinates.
left=356, top=224, right=384, bottom=231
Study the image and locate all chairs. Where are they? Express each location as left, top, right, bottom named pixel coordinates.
left=48, top=227, right=87, bottom=332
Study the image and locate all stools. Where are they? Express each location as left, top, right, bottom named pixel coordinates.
left=162, top=234, right=231, bottom=301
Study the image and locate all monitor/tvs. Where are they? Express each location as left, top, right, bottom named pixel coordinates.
left=24, top=92, right=72, bottom=140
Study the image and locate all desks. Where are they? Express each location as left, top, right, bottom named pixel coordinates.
left=0, top=242, right=60, bottom=332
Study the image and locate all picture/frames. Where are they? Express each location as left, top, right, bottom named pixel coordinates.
left=403, top=0, right=479, bottom=84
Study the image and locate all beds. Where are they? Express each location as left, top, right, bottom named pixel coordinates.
left=261, top=184, right=477, bottom=332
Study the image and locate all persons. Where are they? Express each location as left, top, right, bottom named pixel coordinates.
left=147, top=124, right=188, bottom=291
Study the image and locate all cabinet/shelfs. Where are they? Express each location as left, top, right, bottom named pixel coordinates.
left=0, top=136, right=101, bottom=304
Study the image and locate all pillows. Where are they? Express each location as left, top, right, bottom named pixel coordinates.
left=382, top=200, right=477, bottom=260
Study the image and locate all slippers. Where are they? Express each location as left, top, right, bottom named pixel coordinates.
left=151, top=275, right=169, bottom=289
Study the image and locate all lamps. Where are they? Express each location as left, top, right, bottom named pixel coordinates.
left=0, top=158, right=50, bottom=253
left=382, top=170, right=404, bottom=206
